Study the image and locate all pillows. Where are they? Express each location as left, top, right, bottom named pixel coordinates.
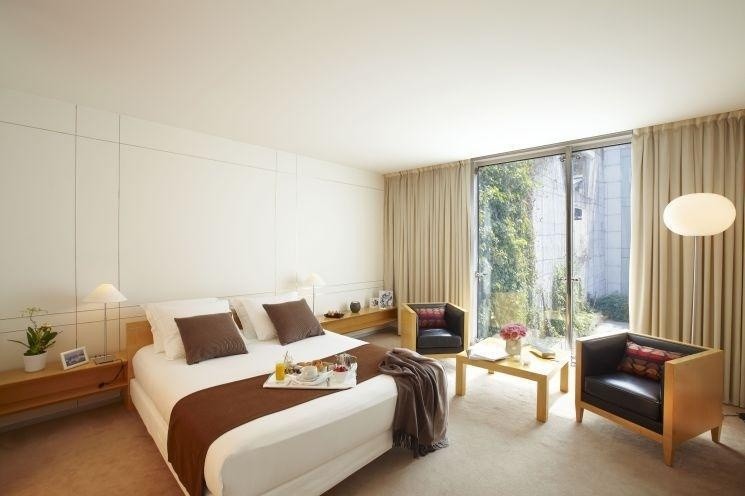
left=173, top=311, right=249, bottom=365
left=231, top=293, right=262, bottom=340
left=138, top=292, right=220, bottom=354
left=414, top=306, right=447, bottom=329
left=616, top=338, right=690, bottom=381
left=147, top=298, right=248, bottom=361
left=262, top=297, right=326, bottom=346
left=235, top=290, right=306, bottom=341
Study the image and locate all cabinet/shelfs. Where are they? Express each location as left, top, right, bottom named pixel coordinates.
left=315, top=303, right=399, bottom=336
left=0, top=351, right=132, bottom=416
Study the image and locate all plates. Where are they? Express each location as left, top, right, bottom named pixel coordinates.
left=292, top=373, right=331, bottom=386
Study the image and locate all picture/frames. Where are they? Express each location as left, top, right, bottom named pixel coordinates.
left=371, top=297, right=379, bottom=307
left=379, top=289, right=394, bottom=308
left=61, top=346, right=89, bottom=370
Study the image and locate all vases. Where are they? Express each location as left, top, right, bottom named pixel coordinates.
left=506, top=336, right=522, bottom=354
left=20, top=352, right=48, bottom=372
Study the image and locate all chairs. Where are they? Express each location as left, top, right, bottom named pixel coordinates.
left=400, top=299, right=470, bottom=358
left=573, top=328, right=725, bottom=466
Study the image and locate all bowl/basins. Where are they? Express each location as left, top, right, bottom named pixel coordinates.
left=301, top=366, right=318, bottom=378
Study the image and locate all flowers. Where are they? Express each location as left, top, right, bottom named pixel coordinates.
left=5, top=304, right=62, bottom=355
left=501, top=322, right=527, bottom=338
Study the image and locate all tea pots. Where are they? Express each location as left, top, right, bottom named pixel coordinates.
left=333, top=353, right=357, bottom=372
left=275, top=361, right=285, bottom=384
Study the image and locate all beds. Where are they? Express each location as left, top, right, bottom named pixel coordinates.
left=124, top=290, right=451, bottom=496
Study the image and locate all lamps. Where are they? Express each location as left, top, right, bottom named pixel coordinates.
left=81, top=281, right=128, bottom=365
left=663, top=191, right=737, bottom=346
